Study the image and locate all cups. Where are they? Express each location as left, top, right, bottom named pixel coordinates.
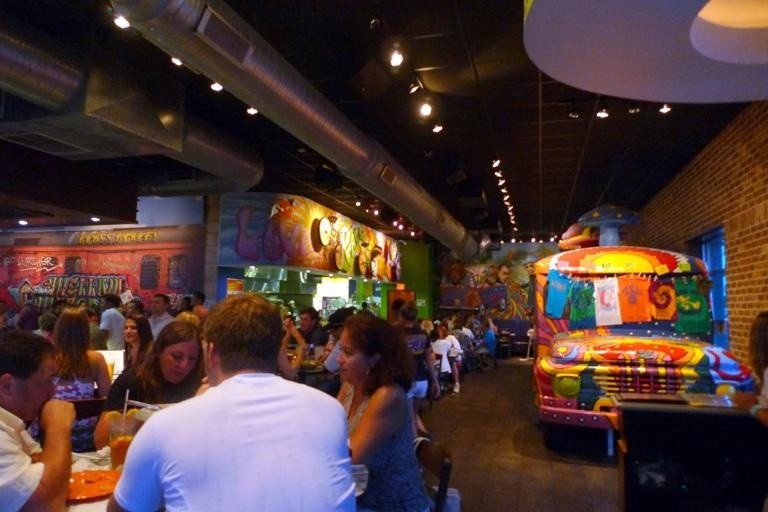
left=107, top=421, right=137, bottom=473
left=105, top=359, right=115, bottom=384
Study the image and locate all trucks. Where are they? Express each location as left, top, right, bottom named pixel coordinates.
left=525, top=246, right=759, bottom=437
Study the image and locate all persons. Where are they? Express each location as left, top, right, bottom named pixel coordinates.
left=495, top=264, right=509, bottom=284
left=1, top=291, right=209, bottom=511
left=106, top=290, right=357, bottom=511
left=278, top=297, right=499, bottom=511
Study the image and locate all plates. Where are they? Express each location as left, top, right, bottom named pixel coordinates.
left=69, top=471, right=124, bottom=504
